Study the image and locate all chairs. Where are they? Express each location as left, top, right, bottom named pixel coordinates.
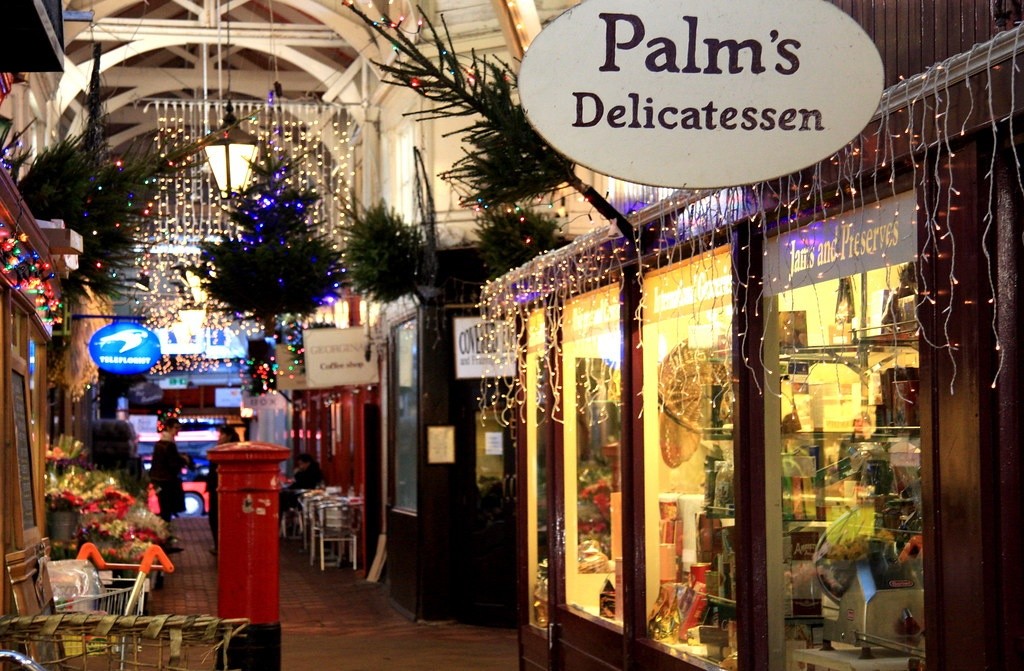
left=284, top=479, right=366, bottom=572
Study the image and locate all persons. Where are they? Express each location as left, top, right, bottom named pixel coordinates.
left=203, top=425, right=239, bottom=553
left=278, top=453, right=324, bottom=525
left=147, top=418, right=189, bottom=549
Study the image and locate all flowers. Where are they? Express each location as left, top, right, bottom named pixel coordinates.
left=39, top=433, right=166, bottom=588
